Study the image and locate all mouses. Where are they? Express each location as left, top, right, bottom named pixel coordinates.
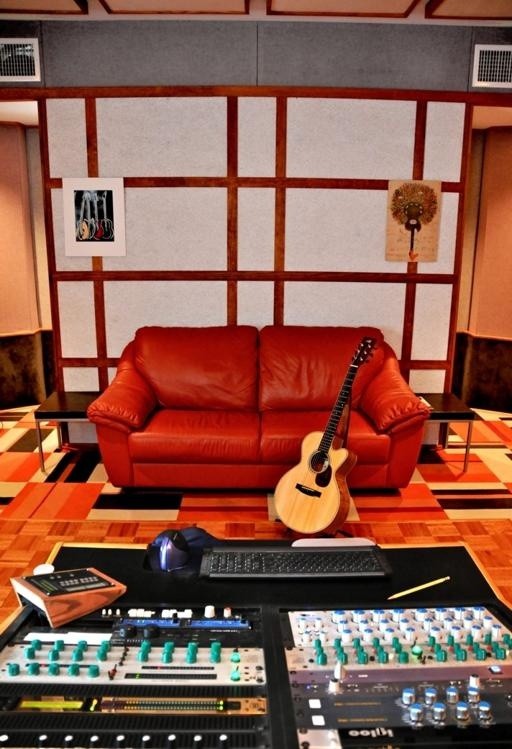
left=142, top=530, right=193, bottom=573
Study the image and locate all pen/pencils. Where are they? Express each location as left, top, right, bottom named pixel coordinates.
left=386, top=576, right=450, bottom=600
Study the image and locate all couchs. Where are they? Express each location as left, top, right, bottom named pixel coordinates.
left=86, top=327, right=434, bottom=496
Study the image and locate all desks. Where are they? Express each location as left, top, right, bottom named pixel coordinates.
left=33, top=389, right=102, bottom=472
left=413, top=393, right=476, bottom=470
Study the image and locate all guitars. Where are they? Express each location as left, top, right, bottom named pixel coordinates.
left=274, top=335, right=377, bottom=535
left=76, top=191, right=114, bottom=241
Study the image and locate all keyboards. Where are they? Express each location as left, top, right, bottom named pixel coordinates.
left=199, top=545, right=393, bottom=578
